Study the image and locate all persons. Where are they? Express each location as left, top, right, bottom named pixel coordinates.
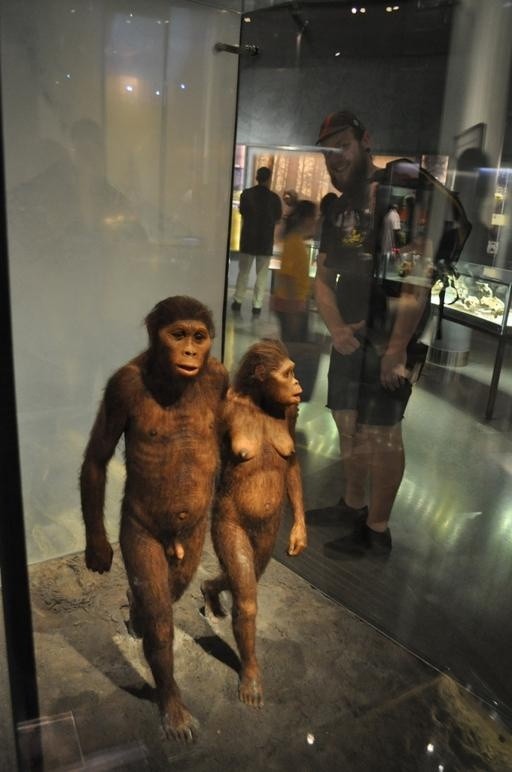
left=231, top=166, right=281, bottom=317
left=275, top=189, right=300, bottom=243
left=312, top=192, right=341, bottom=236
left=274, top=200, right=320, bottom=403
left=304, top=110, right=442, bottom=563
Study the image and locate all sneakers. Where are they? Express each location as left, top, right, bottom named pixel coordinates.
left=252, top=308, right=260, bottom=314
left=232, top=301, right=241, bottom=310
left=323, top=523, right=392, bottom=560
left=306, top=496, right=368, bottom=525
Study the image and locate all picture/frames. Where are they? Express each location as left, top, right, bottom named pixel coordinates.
left=453, top=121, right=488, bottom=157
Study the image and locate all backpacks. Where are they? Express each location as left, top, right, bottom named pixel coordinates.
left=374, top=158, right=472, bottom=296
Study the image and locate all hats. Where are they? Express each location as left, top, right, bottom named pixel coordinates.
left=314, top=111, right=365, bottom=145
